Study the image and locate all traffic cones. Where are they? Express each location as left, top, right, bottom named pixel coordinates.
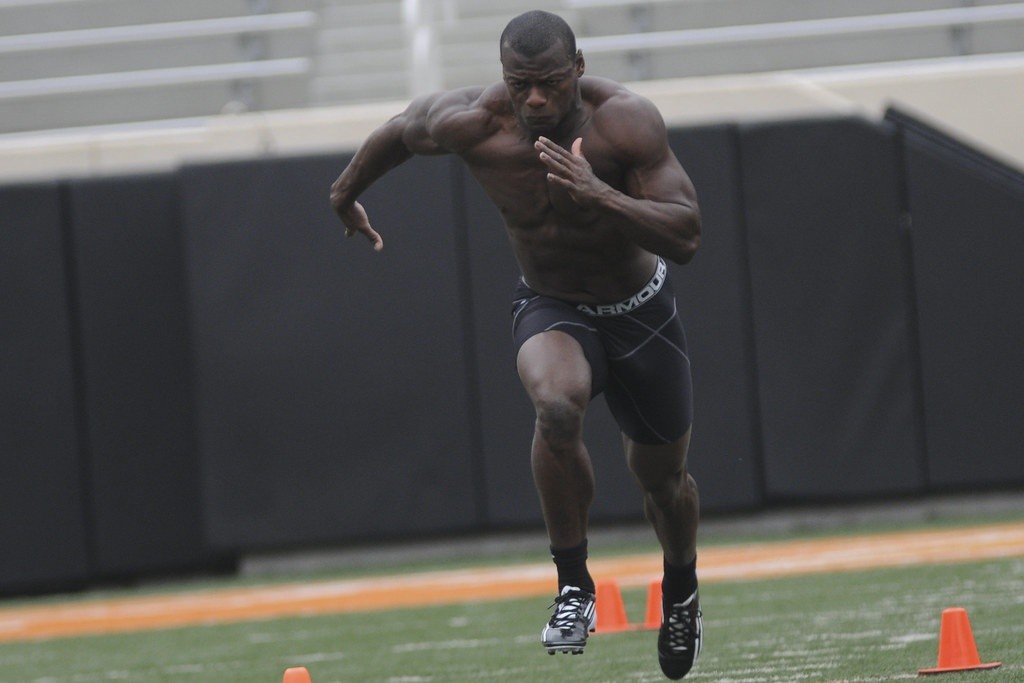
left=589, top=578, right=634, bottom=633
left=282, top=668, right=312, bottom=682
left=637, top=582, right=662, bottom=632
left=917, top=606, right=1005, bottom=677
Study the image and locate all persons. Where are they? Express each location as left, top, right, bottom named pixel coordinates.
left=330, top=10, right=703, bottom=679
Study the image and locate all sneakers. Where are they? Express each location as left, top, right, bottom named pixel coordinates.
left=541, top=585, right=596, bottom=655
left=657, top=587, right=702, bottom=680
